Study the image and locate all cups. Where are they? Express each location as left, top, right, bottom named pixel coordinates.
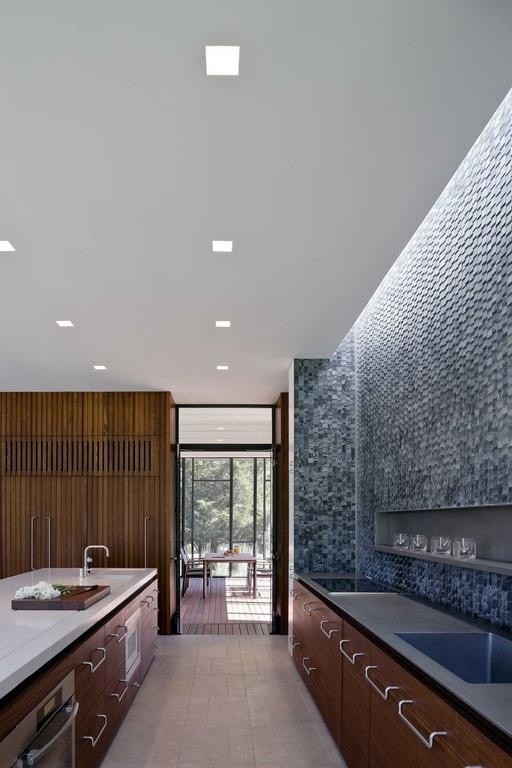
left=430, top=536, right=452, bottom=558
left=408, top=535, right=427, bottom=554
left=453, top=537, right=476, bottom=561
left=233, top=544, right=241, bottom=556
left=217, top=547, right=225, bottom=557
left=392, top=533, right=408, bottom=552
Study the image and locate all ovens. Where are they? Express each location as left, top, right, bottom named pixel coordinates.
left=0, top=668, right=79, bottom=768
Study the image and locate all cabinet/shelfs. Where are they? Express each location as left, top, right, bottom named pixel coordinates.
left=104, top=607, right=127, bottom=750
left=141, top=579, right=160, bottom=683
left=76, top=627, right=108, bottom=767
left=288, top=577, right=447, bottom=765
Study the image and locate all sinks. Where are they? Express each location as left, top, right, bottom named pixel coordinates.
left=81, top=574, right=136, bottom=592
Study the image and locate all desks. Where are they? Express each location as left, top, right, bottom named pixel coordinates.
left=180, top=545, right=272, bottom=599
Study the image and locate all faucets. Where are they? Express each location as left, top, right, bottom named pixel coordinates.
left=83, top=544, right=110, bottom=578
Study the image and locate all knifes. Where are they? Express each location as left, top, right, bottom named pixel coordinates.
left=70, top=584, right=100, bottom=598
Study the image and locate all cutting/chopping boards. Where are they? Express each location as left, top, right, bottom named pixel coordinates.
left=10, top=585, right=112, bottom=611
